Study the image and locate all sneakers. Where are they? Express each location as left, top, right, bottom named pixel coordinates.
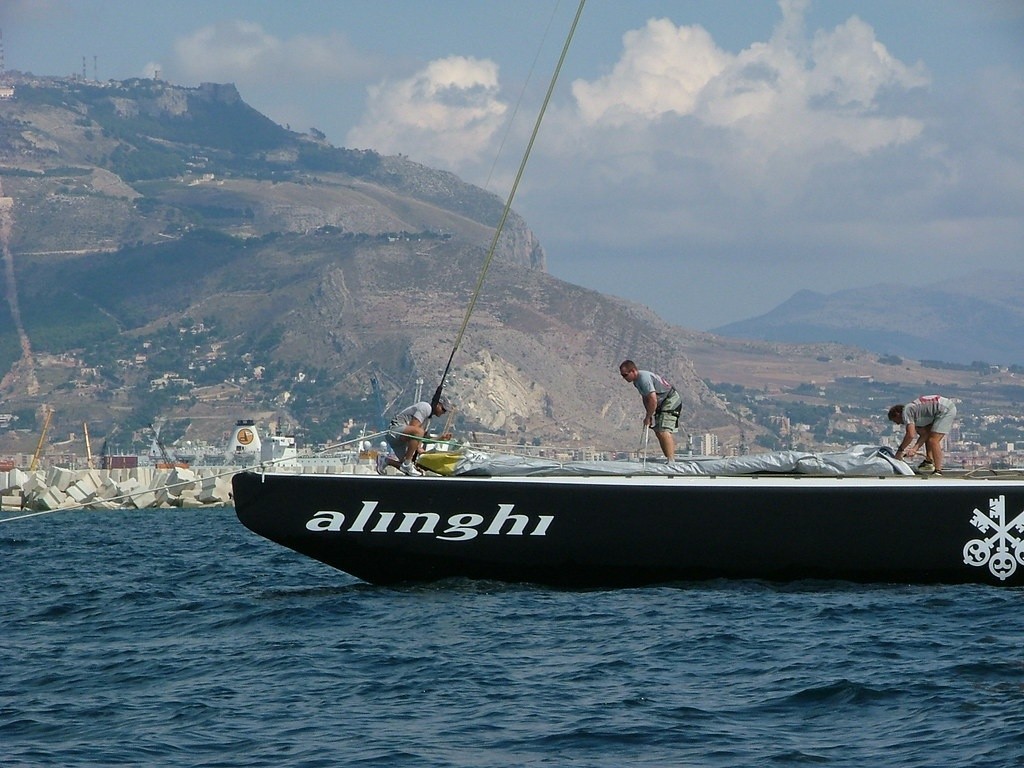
left=932, top=470, right=943, bottom=477
left=376, top=451, right=387, bottom=475
left=400, top=462, right=422, bottom=476
left=914, top=460, right=935, bottom=471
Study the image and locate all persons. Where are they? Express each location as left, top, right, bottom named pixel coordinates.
left=888, top=395, right=957, bottom=476
left=620, top=360, right=682, bottom=465
left=377, top=396, right=452, bottom=477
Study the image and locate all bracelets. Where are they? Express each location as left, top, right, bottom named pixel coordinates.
left=898, top=446, right=904, bottom=452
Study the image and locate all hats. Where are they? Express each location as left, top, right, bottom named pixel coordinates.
left=439, top=395, right=452, bottom=412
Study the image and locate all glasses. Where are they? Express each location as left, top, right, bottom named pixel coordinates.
left=622, top=370, right=632, bottom=377
left=439, top=404, right=446, bottom=412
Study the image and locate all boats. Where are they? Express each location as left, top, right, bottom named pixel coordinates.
left=233, top=460, right=1024, bottom=594
